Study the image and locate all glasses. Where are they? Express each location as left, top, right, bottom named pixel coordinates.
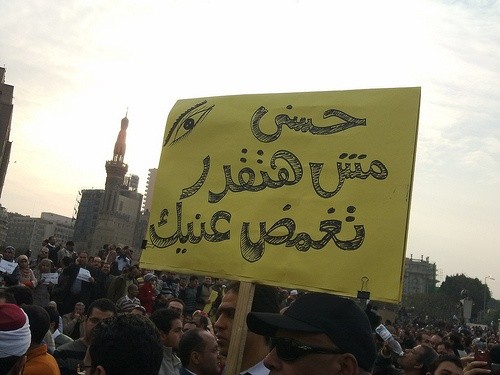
left=72, top=363, right=97, bottom=375
left=268, top=336, right=343, bottom=362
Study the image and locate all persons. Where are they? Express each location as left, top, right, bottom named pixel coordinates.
left=247, top=293, right=377, bottom=375
left=0, top=235, right=500, bottom=375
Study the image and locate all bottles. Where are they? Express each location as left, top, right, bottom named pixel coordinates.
left=375, top=324, right=404, bottom=357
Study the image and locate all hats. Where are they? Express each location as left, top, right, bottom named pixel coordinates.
left=144, top=273, right=156, bottom=282
left=247, top=291, right=378, bottom=373
left=0, top=303, right=31, bottom=358
